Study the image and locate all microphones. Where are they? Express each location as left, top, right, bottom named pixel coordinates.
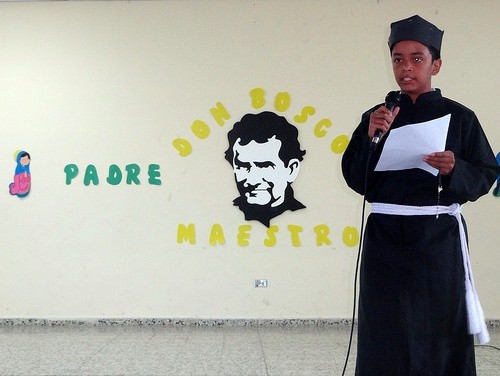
left=370, top=91, right=402, bottom=146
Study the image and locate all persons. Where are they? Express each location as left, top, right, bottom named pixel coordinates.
left=339, top=15, right=498, bottom=376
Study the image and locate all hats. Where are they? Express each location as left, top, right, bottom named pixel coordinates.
left=389, top=14, right=444, bottom=55
